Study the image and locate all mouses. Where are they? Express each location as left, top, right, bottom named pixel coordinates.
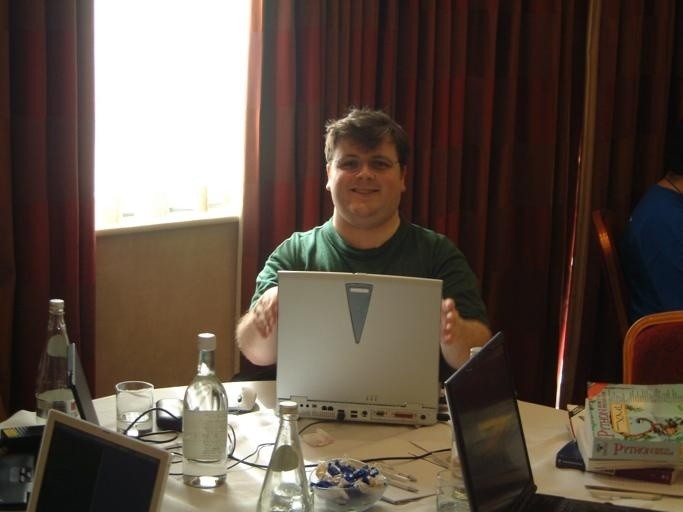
left=226, top=385, right=258, bottom=415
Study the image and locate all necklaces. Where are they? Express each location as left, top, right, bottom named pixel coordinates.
left=662, top=176, right=682, bottom=196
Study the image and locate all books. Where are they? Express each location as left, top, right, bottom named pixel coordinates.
left=554, top=380, right=682, bottom=500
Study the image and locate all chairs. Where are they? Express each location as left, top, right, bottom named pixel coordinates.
left=592, top=206, right=627, bottom=336
left=622, top=310, right=683, bottom=385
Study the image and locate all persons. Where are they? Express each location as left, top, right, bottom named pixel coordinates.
left=616, top=116, right=683, bottom=325
left=232, top=105, right=496, bottom=385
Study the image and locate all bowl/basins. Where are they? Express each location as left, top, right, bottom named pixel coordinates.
left=309, top=476, right=389, bottom=512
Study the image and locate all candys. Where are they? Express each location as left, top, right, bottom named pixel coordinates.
left=310, top=459, right=386, bottom=499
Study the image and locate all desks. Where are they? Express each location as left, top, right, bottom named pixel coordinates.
left=92, top=379, right=683, bottom=512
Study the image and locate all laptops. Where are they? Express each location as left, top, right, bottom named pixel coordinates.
left=443, top=331, right=649, bottom=512
left=276, top=270, right=444, bottom=427
left=1, top=342, right=102, bottom=509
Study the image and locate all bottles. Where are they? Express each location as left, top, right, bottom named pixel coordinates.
left=258, top=400, right=313, bottom=512
left=35, top=298, right=79, bottom=418
left=182, top=332, right=229, bottom=489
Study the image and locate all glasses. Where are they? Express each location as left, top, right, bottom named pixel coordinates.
left=333, top=155, right=398, bottom=172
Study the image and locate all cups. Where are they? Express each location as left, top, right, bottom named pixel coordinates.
left=114, top=380, right=154, bottom=437
left=435, top=467, right=471, bottom=512
